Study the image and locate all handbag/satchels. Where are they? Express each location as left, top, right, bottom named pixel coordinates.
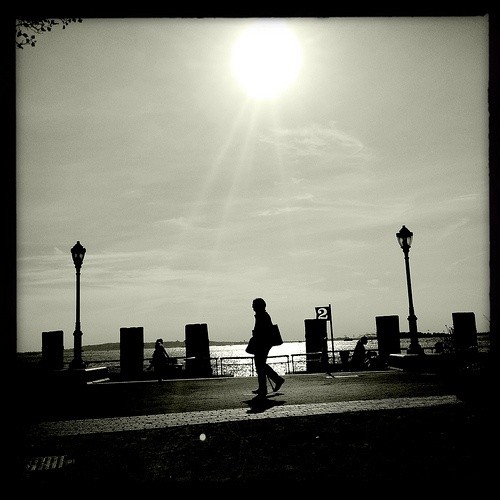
left=272, top=324, right=284, bottom=346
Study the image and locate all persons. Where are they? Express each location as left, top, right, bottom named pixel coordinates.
left=149, top=338, right=169, bottom=380
left=349, top=336, right=377, bottom=372
left=245, top=298, right=284, bottom=400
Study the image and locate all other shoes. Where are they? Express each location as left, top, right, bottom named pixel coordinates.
left=252, top=388, right=267, bottom=394
left=273, top=378, right=285, bottom=392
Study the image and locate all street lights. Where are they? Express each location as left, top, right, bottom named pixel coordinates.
left=396, top=224, right=426, bottom=354
left=70, top=240, right=87, bottom=369
